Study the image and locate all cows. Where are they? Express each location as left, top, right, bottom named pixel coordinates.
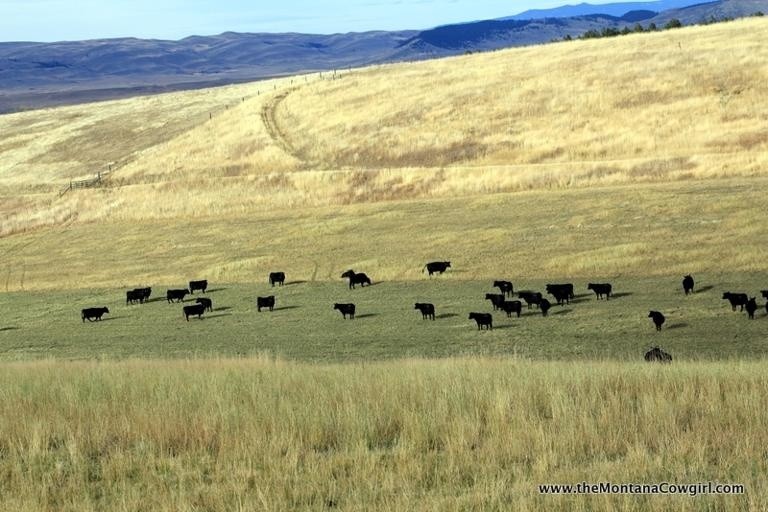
left=468, top=280, right=574, bottom=331
left=257, top=270, right=286, bottom=313
left=414, top=302, right=436, bottom=321
left=333, top=268, right=371, bottom=318
left=587, top=282, right=612, bottom=301
left=722, top=289, right=768, bottom=319
left=125, top=280, right=213, bottom=322
left=647, top=310, right=665, bottom=331
left=682, top=274, right=695, bottom=296
left=81, top=306, right=109, bottom=323
left=421, top=260, right=452, bottom=275
left=643, top=347, right=672, bottom=362
